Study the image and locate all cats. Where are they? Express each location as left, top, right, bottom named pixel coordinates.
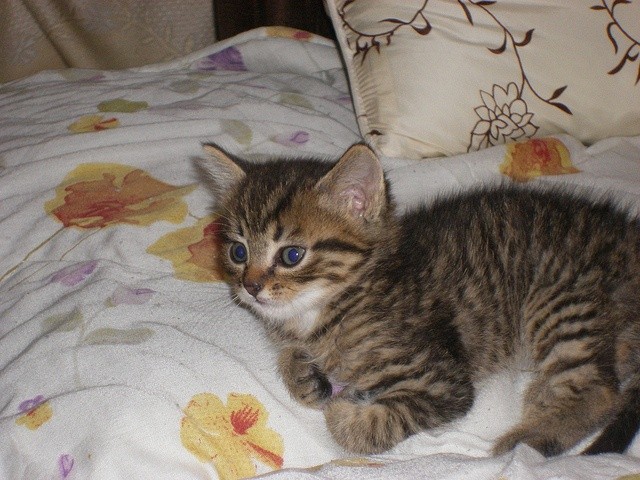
left=169, top=135, right=640, bottom=460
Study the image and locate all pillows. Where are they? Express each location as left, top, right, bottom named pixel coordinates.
left=326, top=0, right=640, bottom=162
